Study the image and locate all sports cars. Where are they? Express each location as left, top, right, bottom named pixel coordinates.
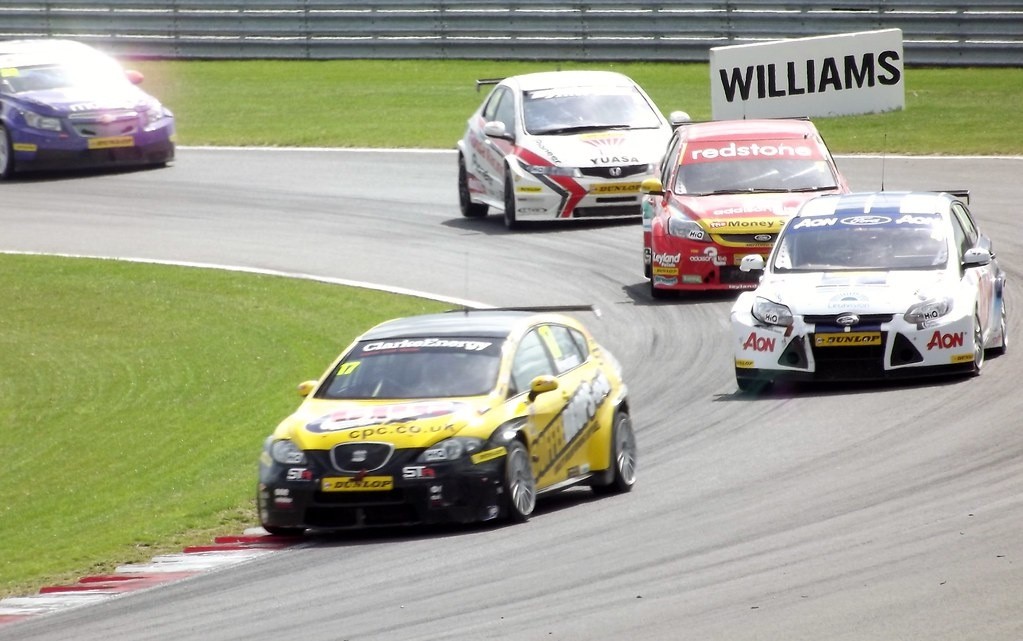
left=0, top=34, right=176, bottom=186
left=456, top=69, right=692, bottom=228
left=635, top=119, right=855, bottom=303
left=730, top=187, right=1011, bottom=393
left=251, top=304, right=637, bottom=537
